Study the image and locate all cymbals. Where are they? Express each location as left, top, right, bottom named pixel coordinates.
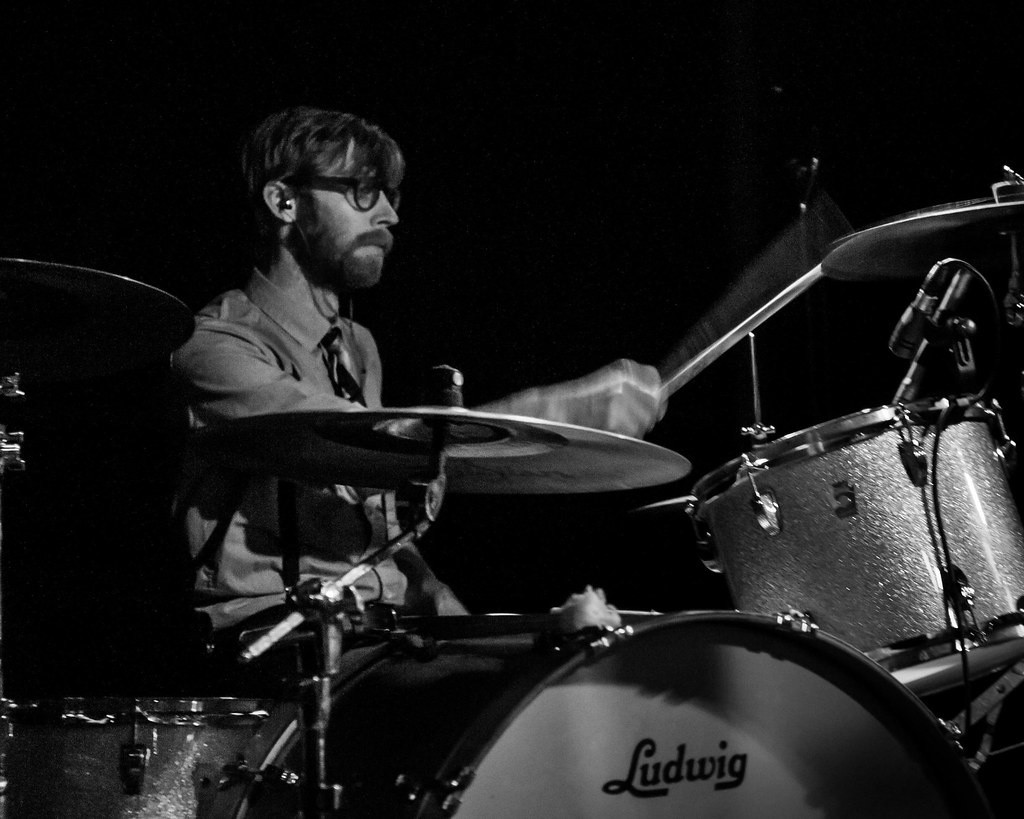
left=182, top=400, right=694, bottom=496
left=821, top=192, right=1024, bottom=283
left=0, top=256, right=198, bottom=389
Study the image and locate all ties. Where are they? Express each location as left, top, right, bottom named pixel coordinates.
left=319, top=328, right=366, bottom=407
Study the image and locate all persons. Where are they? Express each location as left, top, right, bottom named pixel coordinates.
left=162, top=106, right=669, bottom=819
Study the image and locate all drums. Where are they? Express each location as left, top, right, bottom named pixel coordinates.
left=1, top=690, right=278, bottom=819
left=692, top=386, right=1023, bottom=698
left=200, top=604, right=996, bottom=819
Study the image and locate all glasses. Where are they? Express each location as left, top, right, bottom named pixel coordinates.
left=281, top=175, right=401, bottom=212
